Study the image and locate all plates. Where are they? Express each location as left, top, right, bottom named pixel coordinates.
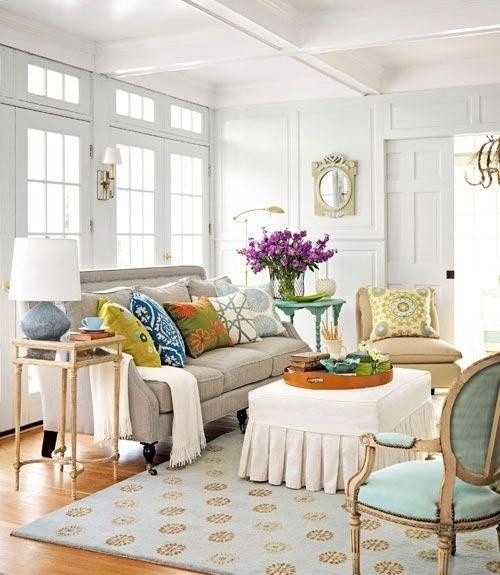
left=79, top=326, right=109, bottom=332
left=287, top=292, right=327, bottom=302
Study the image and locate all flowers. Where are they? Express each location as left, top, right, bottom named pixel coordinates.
left=237, top=226, right=338, bottom=296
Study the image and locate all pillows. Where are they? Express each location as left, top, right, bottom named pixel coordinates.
left=359, top=287, right=439, bottom=341
left=55, top=277, right=290, bottom=368
left=368, top=287, right=441, bottom=342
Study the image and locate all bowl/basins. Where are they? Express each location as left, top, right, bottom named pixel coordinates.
left=320, top=359, right=361, bottom=371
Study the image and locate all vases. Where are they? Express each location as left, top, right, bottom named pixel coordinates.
left=269, top=266, right=304, bottom=299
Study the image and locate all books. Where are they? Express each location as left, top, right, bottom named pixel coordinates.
left=288, top=353, right=331, bottom=374
left=70, top=332, right=116, bottom=342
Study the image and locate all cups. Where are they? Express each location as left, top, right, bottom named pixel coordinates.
left=353, top=362, right=373, bottom=377
left=377, top=361, right=391, bottom=374
left=322, top=339, right=348, bottom=360
left=81, top=317, right=104, bottom=330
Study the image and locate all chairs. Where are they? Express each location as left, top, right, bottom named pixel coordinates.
left=345, top=353, right=500, bottom=575
left=356, top=291, right=463, bottom=395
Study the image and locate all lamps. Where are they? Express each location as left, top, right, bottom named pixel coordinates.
left=9, top=238, right=81, bottom=340
left=96, top=147, right=122, bottom=200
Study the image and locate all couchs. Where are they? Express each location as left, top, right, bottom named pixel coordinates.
left=16, top=265, right=312, bottom=475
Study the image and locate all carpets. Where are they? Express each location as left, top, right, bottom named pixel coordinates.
left=10, top=429, right=500, bottom=575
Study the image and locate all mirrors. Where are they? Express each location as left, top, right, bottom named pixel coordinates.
left=311, top=156, right=357, bottom=217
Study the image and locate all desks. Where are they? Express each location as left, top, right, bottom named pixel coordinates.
left=11, top=335, right=127, bottom=501
left=274, top=299, right=346, bottom=352
